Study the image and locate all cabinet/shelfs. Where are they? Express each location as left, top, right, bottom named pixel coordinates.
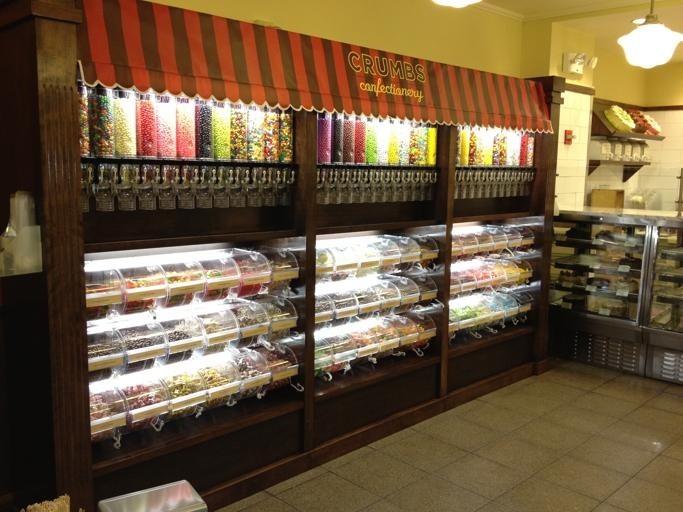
left=37, top=77, right=546, bottom=511
left=545, top=208, right=683, bottom=386
left=588, top=104, right=668, bottom=183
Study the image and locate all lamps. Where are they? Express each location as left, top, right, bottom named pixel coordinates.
left=561, top=49, right=599, bottom=77
left=615, top=0, right=682, bottom=72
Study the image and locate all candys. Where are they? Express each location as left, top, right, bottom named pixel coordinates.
left=422, top=224, right=536, bottom=333
left=293, top=232, right=444, bottom=382
left=317, top=113, right=534, bottom=167
left=81, top=245, right=305, bottom=439
left=80, top=93, right=296, bottom=165
